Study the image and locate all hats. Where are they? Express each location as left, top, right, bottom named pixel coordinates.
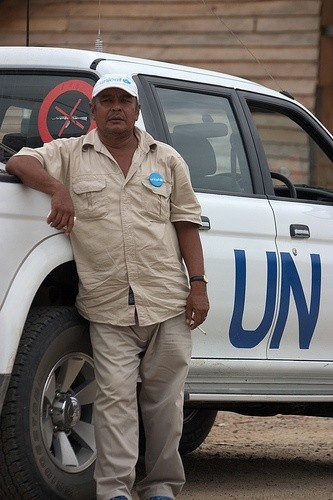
left=92, top=74, right=139, bottom=104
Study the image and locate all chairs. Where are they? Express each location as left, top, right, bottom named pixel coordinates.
left=175, top=133, right=242, bottom=191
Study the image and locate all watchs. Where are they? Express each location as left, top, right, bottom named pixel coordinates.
left=190, top=275, right=208, bottom=283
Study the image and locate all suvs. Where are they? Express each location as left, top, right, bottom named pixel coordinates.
left=1, top=44, right=333, bottom=497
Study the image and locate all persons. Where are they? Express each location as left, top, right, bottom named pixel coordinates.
left=6, top=73, right=210, bottom=500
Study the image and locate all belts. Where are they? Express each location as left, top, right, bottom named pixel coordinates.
left=128, top=293, right=135, bottom=305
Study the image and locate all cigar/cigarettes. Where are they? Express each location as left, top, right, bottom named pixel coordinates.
left=193, top=320, right=207, bottom=336
